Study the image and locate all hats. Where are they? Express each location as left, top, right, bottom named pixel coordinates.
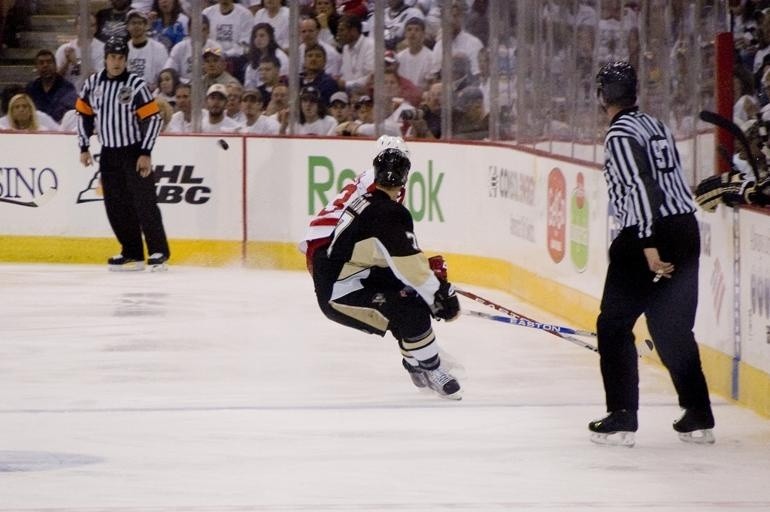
left=299, top=86, right=320, bottom=99
left=354, top=95, right=373, bottom=109
left=330, top=91, right=349, bottom=104
left=206, top=83, right=228, bottom=98
left=451, top=87, right=483, bottom=110
left=127, top=9, right=147, bottom=20
left=383, top=50, right=400, bottom=64
left=241, top=88, right=263, bottom=101
left=202, top=48, right=223, bottom=56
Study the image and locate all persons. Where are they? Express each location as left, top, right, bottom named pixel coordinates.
left=588, top=57, right=714, bottom=433
left=76, top=36, right=170, bottom=266
left=299, top=134, right=449, bottom=297
left=312, top=147, right=461, bottom=395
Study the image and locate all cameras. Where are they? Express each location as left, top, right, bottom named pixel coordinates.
left=402, top=103, right=430, bottom=120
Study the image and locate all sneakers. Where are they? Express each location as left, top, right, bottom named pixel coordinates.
left=588, top=409, right=638, bottom=434
left=424, top=368, right=461, bottom=396
left=107, top=252, right=145, bottom=265
left=672, top=406, right=714, bottom=433
left=147, top=252, right=169, bottom=265
left=402, top=357, right=423, bottom=375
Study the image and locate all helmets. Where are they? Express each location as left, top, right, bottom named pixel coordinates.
left=104, top=36, right=129, bottom=61
left=373, top=133, right=412, bottom=160
left=594, top=59, right=637, bottom=100
left=373, top=148, right=411, bottom=187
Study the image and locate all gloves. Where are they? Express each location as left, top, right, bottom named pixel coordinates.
left=433, top=284, right=459, bottom=320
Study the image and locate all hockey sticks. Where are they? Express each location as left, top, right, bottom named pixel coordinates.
left=459, top=310, right=653, bottom=357
left=699, top=110, right=758, bottom=179
